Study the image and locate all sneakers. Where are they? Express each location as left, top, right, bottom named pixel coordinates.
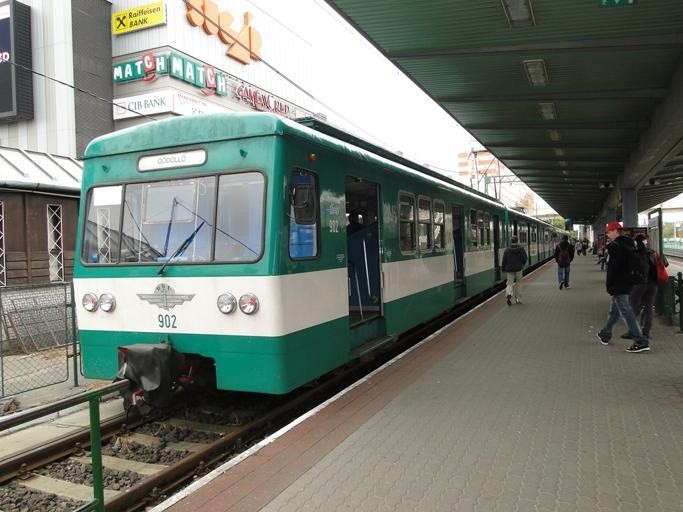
left=625, top=341, right=651, bottom=354
left=621, top=331, right=649, bottom=339
left=596, top=330, right=608, bottom=346
left=506, top=293, right=522, bottom=305
left=558, top=281, right=570, bottom=290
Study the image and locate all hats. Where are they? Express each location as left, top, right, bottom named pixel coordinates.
left=604, top=221, right=621, bottom=232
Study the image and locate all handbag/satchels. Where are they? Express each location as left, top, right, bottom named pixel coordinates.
left=654, top=252, right=668, bottom=283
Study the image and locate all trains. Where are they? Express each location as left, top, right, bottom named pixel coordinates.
left=73, top=111, right=581, bottom=421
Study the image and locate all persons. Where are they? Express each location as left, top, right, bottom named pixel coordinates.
left=345, top=212, right=364, bottom=234
left=570, top=238, right=589, bottom=257
left=593, top=222, right=669, bottom=353
left=554, top=235, right=574, bottom=290
left=502, top=236, right=527, bottom=305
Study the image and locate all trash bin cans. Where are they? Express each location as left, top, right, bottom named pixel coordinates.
left=655, top=276, right=675, bottom=316
